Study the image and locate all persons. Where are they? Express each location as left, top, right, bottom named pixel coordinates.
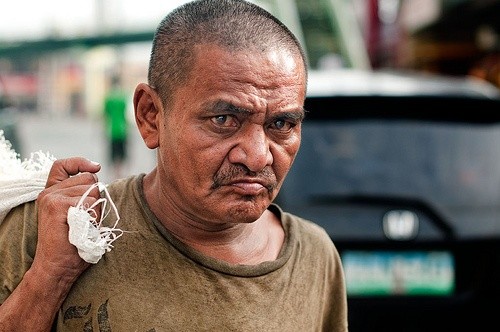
left=0, top=1, right=351, bottom=331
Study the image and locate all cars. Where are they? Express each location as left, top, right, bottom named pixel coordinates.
left=279, top=68, right=499, bottom=331
